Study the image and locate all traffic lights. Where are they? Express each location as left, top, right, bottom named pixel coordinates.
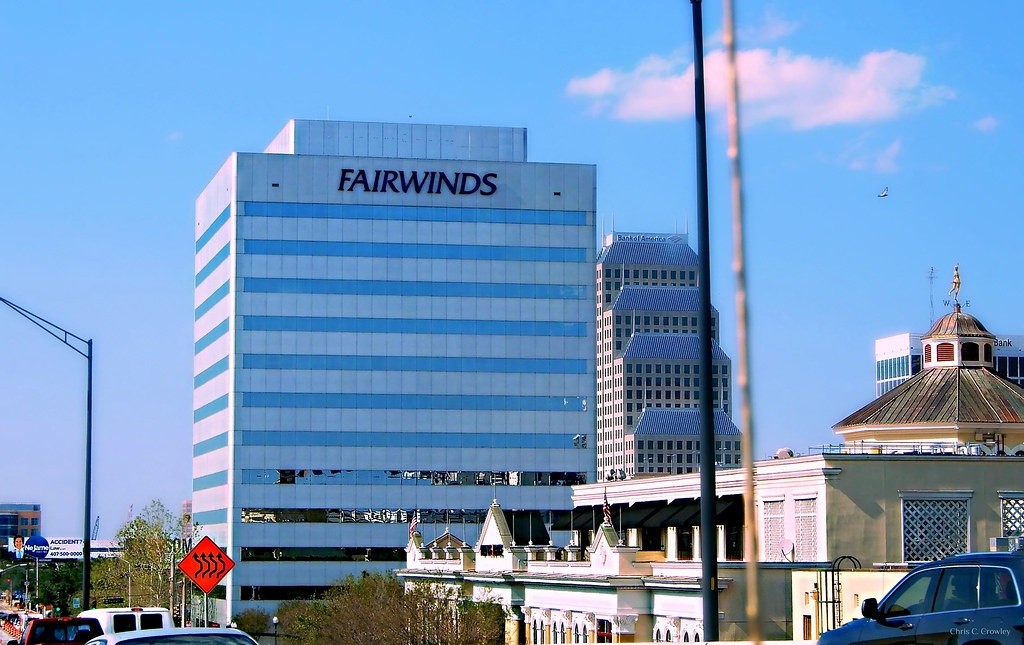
left=92, top=601, right=96, bottom=610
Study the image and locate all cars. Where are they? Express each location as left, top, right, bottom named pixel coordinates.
left=82, top=626, right=258, bottom=645
left=816, top=550, right=1024, bottom=645
left=0, top=611, right=41, bottom=629
left=6, top=617, right=104, bottom=645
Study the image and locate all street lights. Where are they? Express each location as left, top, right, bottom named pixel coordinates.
left=271, top=616, right=280, bottom=644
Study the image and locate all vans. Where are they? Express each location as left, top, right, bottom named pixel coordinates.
left=76, top=606, right=175, bottom=633
